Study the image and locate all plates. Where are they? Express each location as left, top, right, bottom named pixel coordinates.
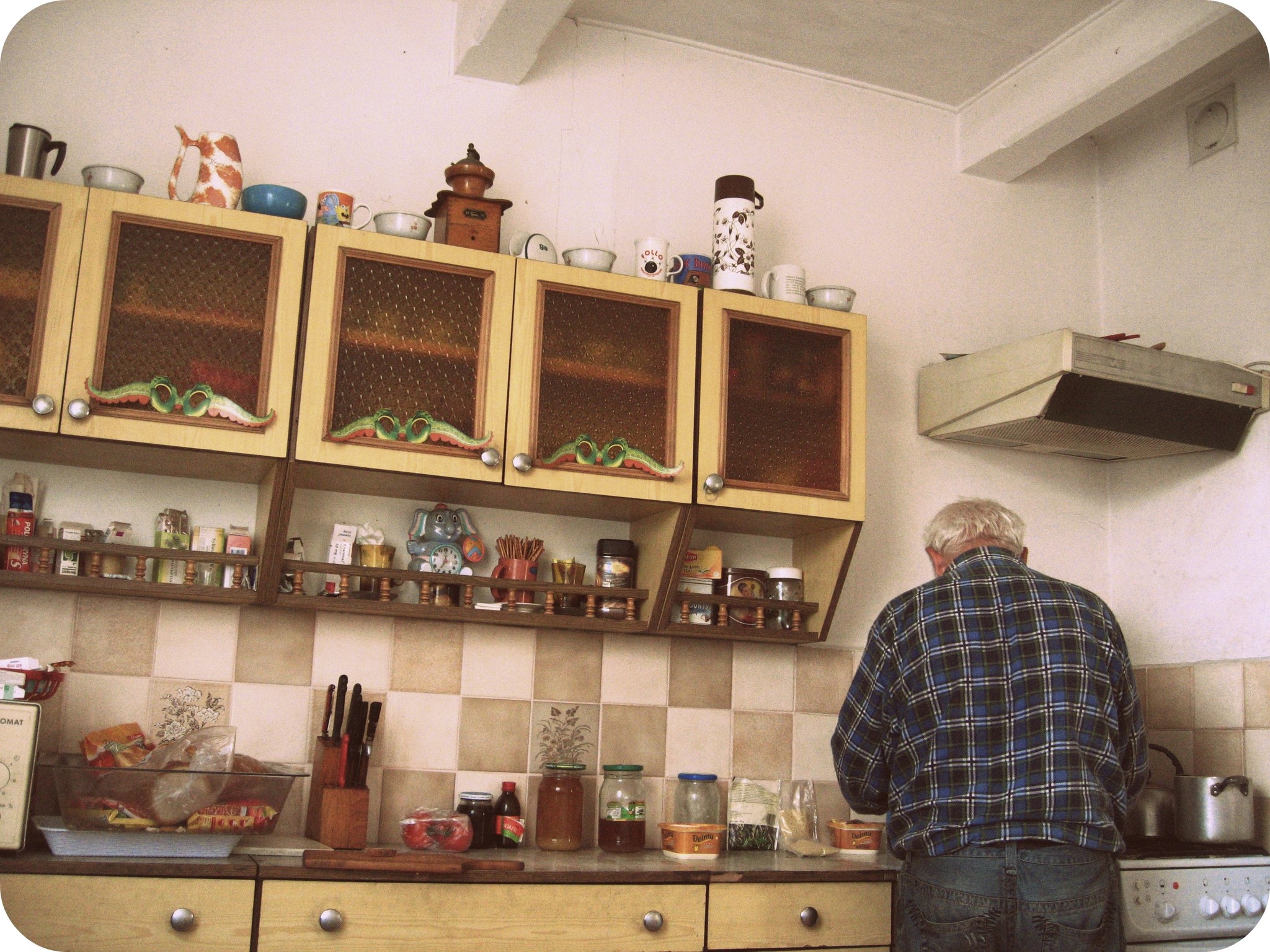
left=494, top=602, right=544, bottom=613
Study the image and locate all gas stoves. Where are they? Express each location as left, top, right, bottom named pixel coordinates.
left=1114, top=840, right=1269, bottom=944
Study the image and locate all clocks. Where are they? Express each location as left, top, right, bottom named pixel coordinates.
left=398, top=504, right=485, bottom=607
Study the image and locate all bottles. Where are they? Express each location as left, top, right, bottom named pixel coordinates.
left=712, top=175, right=764, bottom=296
left=535, top=763, right=586, bottom=850
left=673, top=773, right=722, bottom=825
left=456, top=792, right=494, bottom=849
left=766, top=567, right=803, bottom=631
left=595, top=538, right=636, bottom=619
left=598, top=765, right=645, bottom=854
left=494, top=782, right=521, bottom=848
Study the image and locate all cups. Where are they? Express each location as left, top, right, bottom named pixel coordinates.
left=5, top=123, right=67, bottom=180
left=359, top=544, right=396, bottom=600
left=490, top=558, right=539, bottom=607
left=634, top=236, right=683, bottom=282
left=315, top=191, right=372, bottom=230
left=761, top=264, right=807, bottom=305
left=551, top=562, right=586, bottom=615
left=667, top=253, right=713, bottom=289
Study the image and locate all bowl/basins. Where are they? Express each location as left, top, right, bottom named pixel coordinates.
left=373, top=210, right=433, bottom=241
left=805, top=285, right=856, bottom=313
left=562, top=246, right=618, bottom=273
left=241, top=184, right=309, bottom=220
left=81, top=163, right=145, bottom=194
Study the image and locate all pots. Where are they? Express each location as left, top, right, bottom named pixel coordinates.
left=1172, top=773, right=1256, bottom=846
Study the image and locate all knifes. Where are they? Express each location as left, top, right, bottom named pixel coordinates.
left=320, top=674, right=383, bottom=787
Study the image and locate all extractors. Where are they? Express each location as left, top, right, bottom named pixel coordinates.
left=915, top=328, right=1270, bottom=462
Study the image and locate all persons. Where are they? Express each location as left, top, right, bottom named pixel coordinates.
left=830, top=498, right=1151, bottom=952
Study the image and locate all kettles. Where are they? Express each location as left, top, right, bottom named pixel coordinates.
left=1125, top=743, right=1184, bottom=839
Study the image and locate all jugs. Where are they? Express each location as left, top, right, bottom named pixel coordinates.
left=168, top=124, right=243, bottom=209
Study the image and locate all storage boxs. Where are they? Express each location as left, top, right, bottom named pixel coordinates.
left=286, top=537, right=305, bottom=561
left=826, top=821, right=885, bottom=851
left=55, top=521, right=133, bottom=576
left=0, top=657, right=39, bottom=700
left=35, top=751, right=310, bottom=834
left=151, top=524, right=252, bottom=588
left=665, top=550, right=722, bottom=625
left=321, top=524, right=358, bottom=593
left=3, top=509, right=37, bottom=572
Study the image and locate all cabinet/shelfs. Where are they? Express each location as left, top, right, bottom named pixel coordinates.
left=707, top=880, right=895, bottom=951
left=253, top=878, right=708, bottom=952
left=648, top=287, right=868, bottom=641
left=0, top=873, right=256, bottom=952
left=265, top=224, right=697, bottom=633
left=0, top=175, right=308, bottom=606
